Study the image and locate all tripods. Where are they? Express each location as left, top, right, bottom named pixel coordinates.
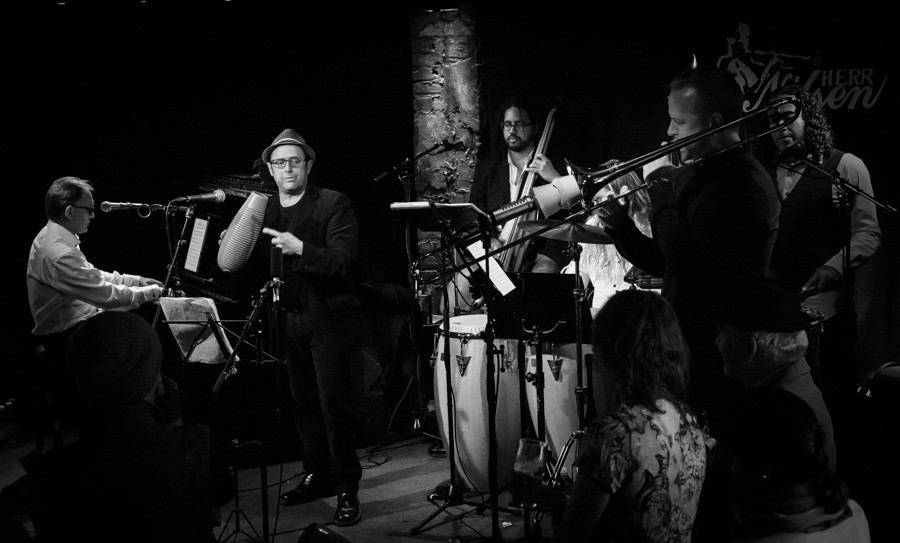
left=162, top=311, right=288, bottom=543
left=366, top=138, right=599, bottom=535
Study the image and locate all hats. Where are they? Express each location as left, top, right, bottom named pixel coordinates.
left=67, top=311, right=162, bottom=404
left=262, top=129, right=316, bottom=164
left=704, top=286, right=816, bottom=333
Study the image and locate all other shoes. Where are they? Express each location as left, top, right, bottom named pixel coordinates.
left=436, top=481, right=468, bottom=495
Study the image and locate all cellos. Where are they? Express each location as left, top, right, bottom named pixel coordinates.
left=376, top=73, right=569, bottom=313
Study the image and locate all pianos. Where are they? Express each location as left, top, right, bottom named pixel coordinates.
left=149, top=162, right=417, bottom=461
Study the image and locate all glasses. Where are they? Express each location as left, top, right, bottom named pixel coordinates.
left=64, top=204, right=95, bottom=215
left=500, top=121, right=532, bottom=130
left=270, top=158, right=309, bottom=167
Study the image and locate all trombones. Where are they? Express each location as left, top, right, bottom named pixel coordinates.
left=409, top=95, right=805, bottom=285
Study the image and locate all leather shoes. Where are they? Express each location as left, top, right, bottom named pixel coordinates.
left=333, top=489, right=362, bottom=527
left=279, top=472, right=337, bottom=505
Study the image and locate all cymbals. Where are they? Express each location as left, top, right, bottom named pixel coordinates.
left=418, top=269, right=439, bottom=273
left=518, top=219, right=617, bottom=244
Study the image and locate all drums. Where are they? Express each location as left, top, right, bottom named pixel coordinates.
left=433, top=312, right=523, bottom=495
left=520, top=339, right=592, bottom=480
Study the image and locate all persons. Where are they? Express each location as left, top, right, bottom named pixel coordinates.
left=218, top=128, right=363, bottom=527
left=27, top=175, right=214, bottom=543
left=479, top=68, right=900, bottom=543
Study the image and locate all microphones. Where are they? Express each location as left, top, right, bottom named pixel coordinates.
left=437, top=138, right=468, bottom=153
left=459, top=196, right=536, bottom=233
left=175, top=190, right=225, bottom=203
left=100, top=201, right=150, bottom=212
left=270, top=246, right=283, bottom=309
left=777, top=141, right=805, bottom=159
